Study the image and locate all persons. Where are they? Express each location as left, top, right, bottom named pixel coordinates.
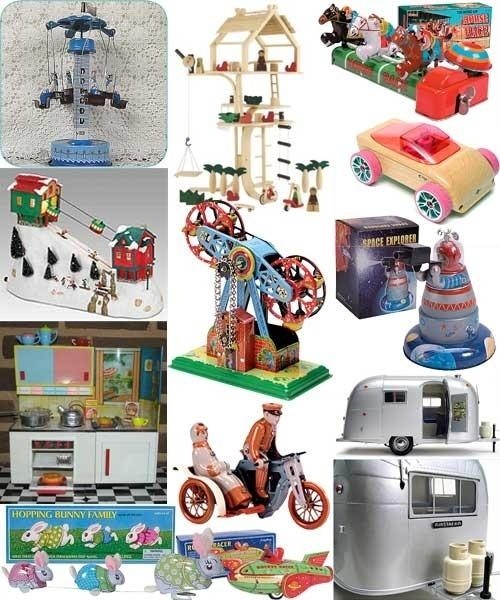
left=179, top=401, right=331, bottom=530
